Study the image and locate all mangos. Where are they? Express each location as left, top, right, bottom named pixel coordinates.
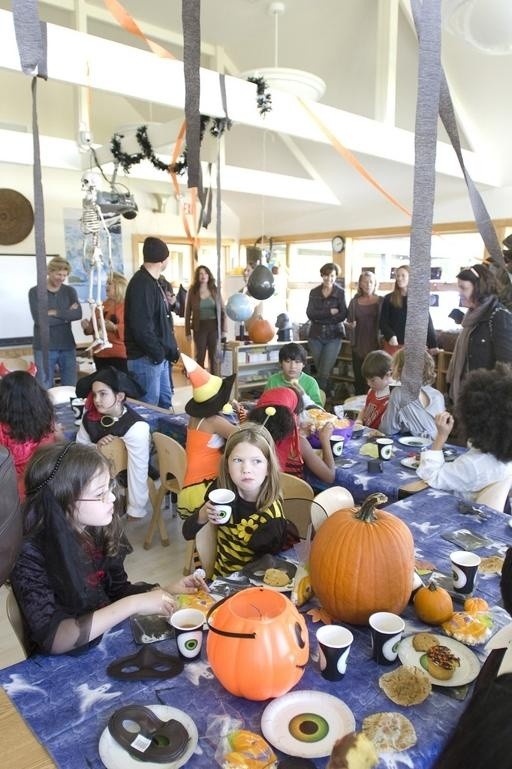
left=222, top=728, right=278, bottom=768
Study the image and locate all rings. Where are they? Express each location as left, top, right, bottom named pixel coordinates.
left=192, top=569, right=206, bottom=580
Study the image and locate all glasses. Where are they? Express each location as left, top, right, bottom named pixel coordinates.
left=75, top=479, right=116, bottom=503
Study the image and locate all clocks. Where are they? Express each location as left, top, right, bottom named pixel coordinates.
left=331, top=235, right=346, bottom=254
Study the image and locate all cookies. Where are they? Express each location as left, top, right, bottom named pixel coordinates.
left=413, top=632, right=441, bottom=652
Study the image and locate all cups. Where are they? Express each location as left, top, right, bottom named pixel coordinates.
left=315, top=623, right=354, bottom=681
left=409, top=572, right=425, bottom=604
left=330, top=434, right=345, bottom=457
left=376, top=438, right=393, bottom=461
left=170, top=607, right=206, bottom=661
left=71, top=402, right=85, bottom=421
left=449, top=551, right=481, bottom=594
left=207, top=487, right=236, bottom=525
left=368, top=611, right=405, bottom=665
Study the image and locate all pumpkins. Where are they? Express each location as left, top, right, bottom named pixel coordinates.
left=465, top=596, right=489, bottom=612
left=413, top=582, right=454, bottom=625
left=307, top=491, right=416, bottom=626
left=247, top=312, right=276, bottom=344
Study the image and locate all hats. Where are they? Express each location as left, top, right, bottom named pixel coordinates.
left=177, top=348, right=236, bottom=417
left=143, top=237, right=169, bottom=262
left=256, top=387, right=301, bottom=456
left=76, top=366, right=147, bottom=398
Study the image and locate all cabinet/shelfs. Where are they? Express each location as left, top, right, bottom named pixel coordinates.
left=219, top=342, right=457, bottom=411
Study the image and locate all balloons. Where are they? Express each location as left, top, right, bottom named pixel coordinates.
left=226, top=260, right=275, bottom=346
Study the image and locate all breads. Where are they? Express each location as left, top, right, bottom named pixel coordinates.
left=264, top=568, right=291, bottom=587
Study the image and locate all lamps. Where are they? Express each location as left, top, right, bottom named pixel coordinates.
left=241, top=1, right=325, bottom=105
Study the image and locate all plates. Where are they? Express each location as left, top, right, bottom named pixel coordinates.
left=400, top=455, right=419, bottom=469
left=398, top=436, right=432, bottom=448
left=97, top=703, right=199, bottom=768
left=251, top=564, right=307, bottom=592
left=397, top=633, right=481, bottom=687
left=261, top=689, right=357, bottom=760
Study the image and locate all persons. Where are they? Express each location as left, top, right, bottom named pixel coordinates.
left=15, top=443, right=209, bottom=656
left=250, top=344, right=336, bottom=485
left=418, top=265, right=512, bottom=517
left=30, top=258, right=82, bottom=389
left=81, top=273, right=130, bottom=373
left=177, top=351, right=249, bottom=517
left=183, top=423, right=284, bottom=583
left=185, top=266, right=226, bottom=375
left=1, top=371, right=57, bottom=500
left=74, top=369, right=160, bottom=523
left=302, top=254, right=446, bottom=437
left=124, top=237, right=181, bottom=408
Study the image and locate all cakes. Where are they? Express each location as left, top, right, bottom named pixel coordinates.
left=443, top=611, right=498, bottom=645
left=420, top=644, right=461, bottom=679
